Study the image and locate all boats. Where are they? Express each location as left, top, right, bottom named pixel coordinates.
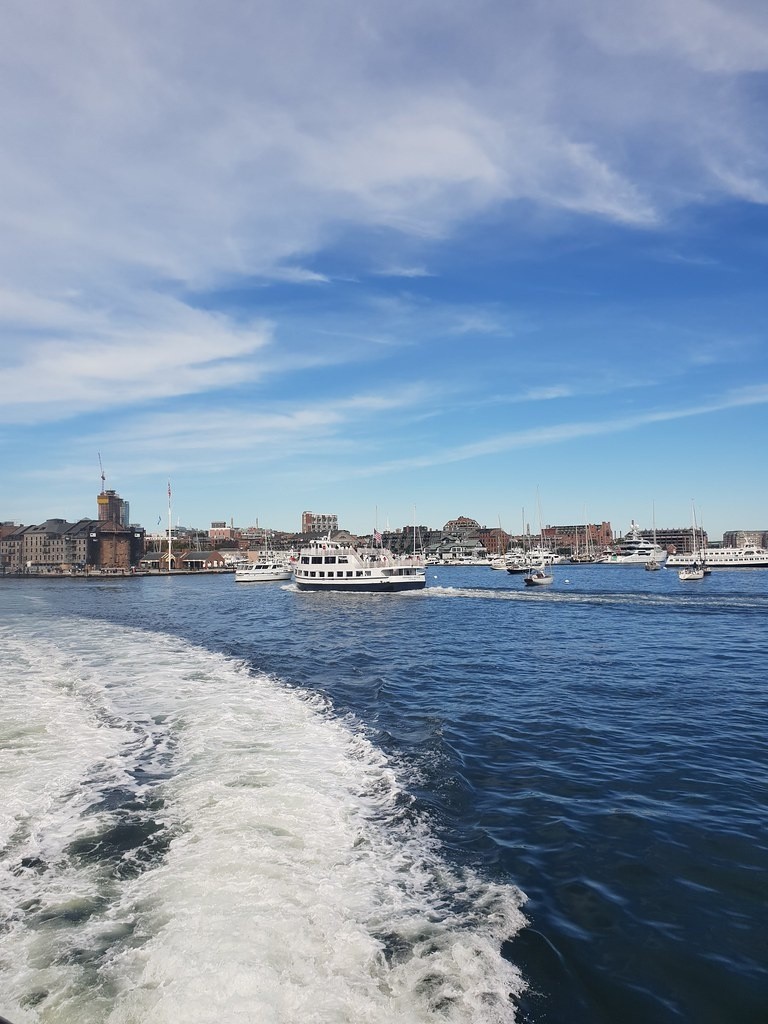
left=291, top=539, right=426, bottom=594
left=596, top=520, right=667, bottom=563
left=666, top=542, right=768, bottom=568
left=526, top=568, right=554, bottom=586
left=235, top=528, right=291, bottom=582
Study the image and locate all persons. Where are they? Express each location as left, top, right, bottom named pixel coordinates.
left=392, top=555, right=395, bottom=560
left=370, top=556, right=386, bottom=561
left=290, top=556, right=299, bottom=561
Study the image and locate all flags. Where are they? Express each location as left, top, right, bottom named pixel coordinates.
left=374, top=529, right=382, bottom=542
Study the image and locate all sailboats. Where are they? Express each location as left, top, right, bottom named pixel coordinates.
left=645, top=498, right=661, bottom=571
left=678, top=497, right=711, bottom=580
left=411, top=507, right=598, bottom=574
